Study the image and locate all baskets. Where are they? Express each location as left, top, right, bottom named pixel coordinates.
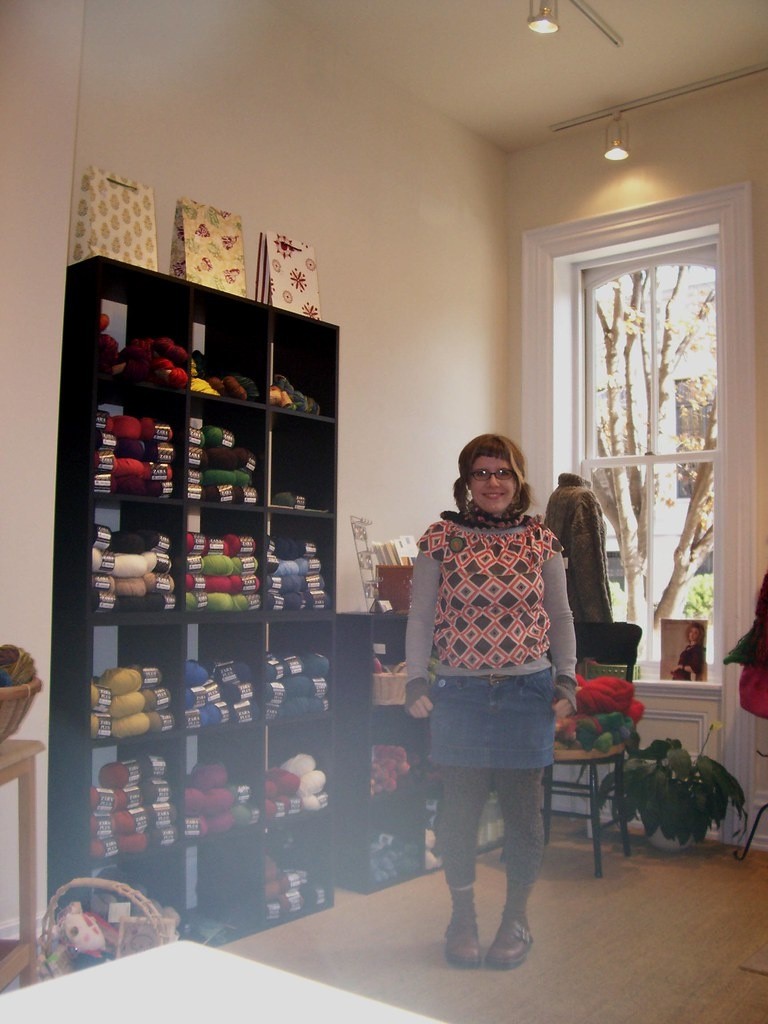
left=375, top=565, right=414, bottom=615
left=371, top=661, right=407, bottom=706
left=0, top=677, right=43, bottom=743
left=37, top=878, right=175, bottom=981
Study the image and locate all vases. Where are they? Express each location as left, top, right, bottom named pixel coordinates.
left=647, top=826, right=694, bottom=853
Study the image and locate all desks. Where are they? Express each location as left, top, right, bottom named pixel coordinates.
left=0, top=740, right=45, bottom=995
left=0, top=941, right=441, bottom=1024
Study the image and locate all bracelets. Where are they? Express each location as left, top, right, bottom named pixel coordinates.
left=682, top=665, right=685, bottom=670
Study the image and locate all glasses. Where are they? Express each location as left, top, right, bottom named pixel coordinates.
left=470, top=468, right=515, bottom=481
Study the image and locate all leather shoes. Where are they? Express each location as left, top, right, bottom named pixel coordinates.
left=486, top=917, right=532, bottom=966
left=445, top=921, right=481, bottom=966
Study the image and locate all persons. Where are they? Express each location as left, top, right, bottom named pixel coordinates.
left=670, top=623, right=706, bottom=679
left=55, top=901, right=115, bottom=972
left=406, top=434, right=577, bottom=969
left=546, top=473, right=613, bottom=623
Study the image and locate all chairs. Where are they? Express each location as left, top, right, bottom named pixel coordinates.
left=499, top=622, right=642, bottom=877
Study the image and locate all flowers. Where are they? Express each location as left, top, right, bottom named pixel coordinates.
left=596, top=719, right=750, bottom=844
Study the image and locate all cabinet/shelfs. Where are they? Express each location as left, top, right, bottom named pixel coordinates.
left=336, top=613, right=504, bottom=895
left=48, top=255, right=336, bottom=970
